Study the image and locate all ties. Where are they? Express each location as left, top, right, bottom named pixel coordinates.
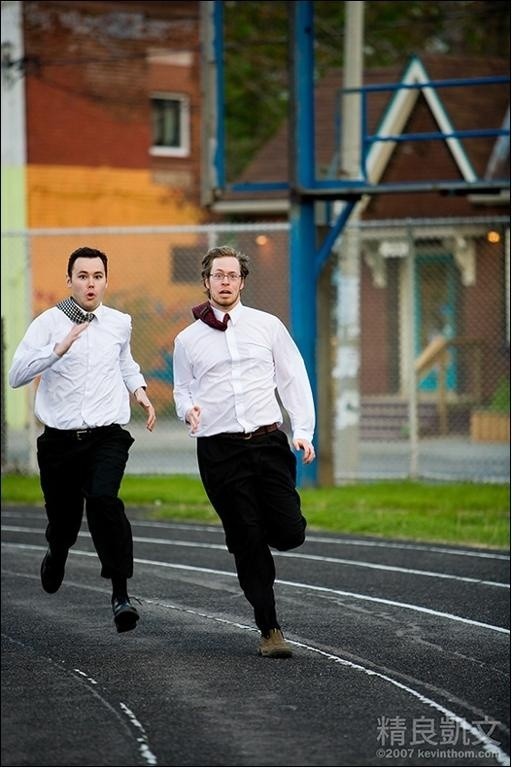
left=57, top=296, right=94, bottom=324
left=191, top=302, right=230, bottom=331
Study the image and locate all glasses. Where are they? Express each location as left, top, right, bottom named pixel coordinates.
left=210, top=273, right=241, bottom=281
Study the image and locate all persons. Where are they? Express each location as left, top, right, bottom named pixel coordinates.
left=9, top=246, right=158, bottom=632
left=173, top=246, right=316, bottom=657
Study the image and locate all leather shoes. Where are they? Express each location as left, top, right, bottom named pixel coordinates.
left=42, top=548, right=66, bottom=593
left=111, top=596, right=139, bottom=633
left=257, top=628, right=291, bottom=658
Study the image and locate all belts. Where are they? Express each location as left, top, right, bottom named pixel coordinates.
left=221, top=426, right=277, bottom=440
left=58, top=428, right=106, bottom=440
